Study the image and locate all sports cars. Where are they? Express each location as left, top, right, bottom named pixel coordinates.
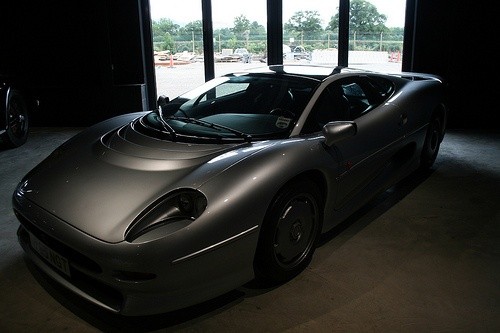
left=11, top=64, right=446, bottom=315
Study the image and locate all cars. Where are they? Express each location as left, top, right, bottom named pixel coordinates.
left=0, top=68, right=56, bottom=147
left=150, top=42, right=308, bottom=65
